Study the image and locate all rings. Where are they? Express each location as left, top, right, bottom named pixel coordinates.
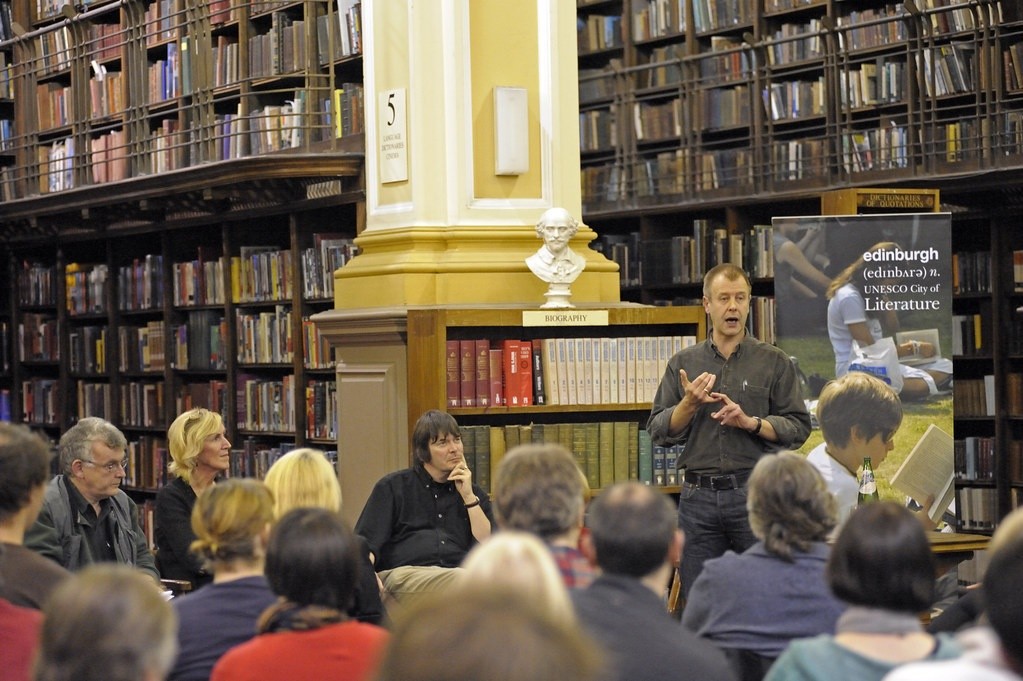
left=703, top=388, right=710, bottom=394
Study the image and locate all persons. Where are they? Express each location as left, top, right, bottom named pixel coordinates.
left=646, top=263, right=811, bottom=624
left=774, top=218, right=832, bottom=297
left=525, top=208, right=585, bottom=283
left=0, top=408, right=1023, bottom=681
left=829, top=241, right=953, bottom=397
left=806, top=370, right=942, bottom=540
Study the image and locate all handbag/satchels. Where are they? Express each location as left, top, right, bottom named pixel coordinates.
left=848, top=336, right=903, bottom=397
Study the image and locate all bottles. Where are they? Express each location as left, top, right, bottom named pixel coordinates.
left=858, top=456, right=880, bottom=505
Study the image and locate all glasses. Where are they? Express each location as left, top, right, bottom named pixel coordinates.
left=75, top=456, right=130, bottom=475
left=867, top=426, right=897, bottom=445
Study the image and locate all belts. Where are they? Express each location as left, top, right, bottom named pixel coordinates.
left=684, top=471, right=749, bottom=490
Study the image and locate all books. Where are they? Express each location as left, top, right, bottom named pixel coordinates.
left=890, top=425, right=954, bottom=523
left=576, top=0, right=1023, bottom=614
left=897, top=329, right=942, bottom=366
left=0, top=233, right=361, bottom=550
left=0, top=0, right=363, bottom=202
left=446, top=336, right=695, bottom=492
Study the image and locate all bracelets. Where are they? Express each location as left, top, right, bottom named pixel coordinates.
left=911, top=341, right=920, bottom=356
left=464, top=496, right=480, bottom=507
left=747, top=415, right=762, bottom=437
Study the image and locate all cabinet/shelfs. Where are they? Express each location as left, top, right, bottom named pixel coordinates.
left=0, top=0, right=1023, bottom=623
